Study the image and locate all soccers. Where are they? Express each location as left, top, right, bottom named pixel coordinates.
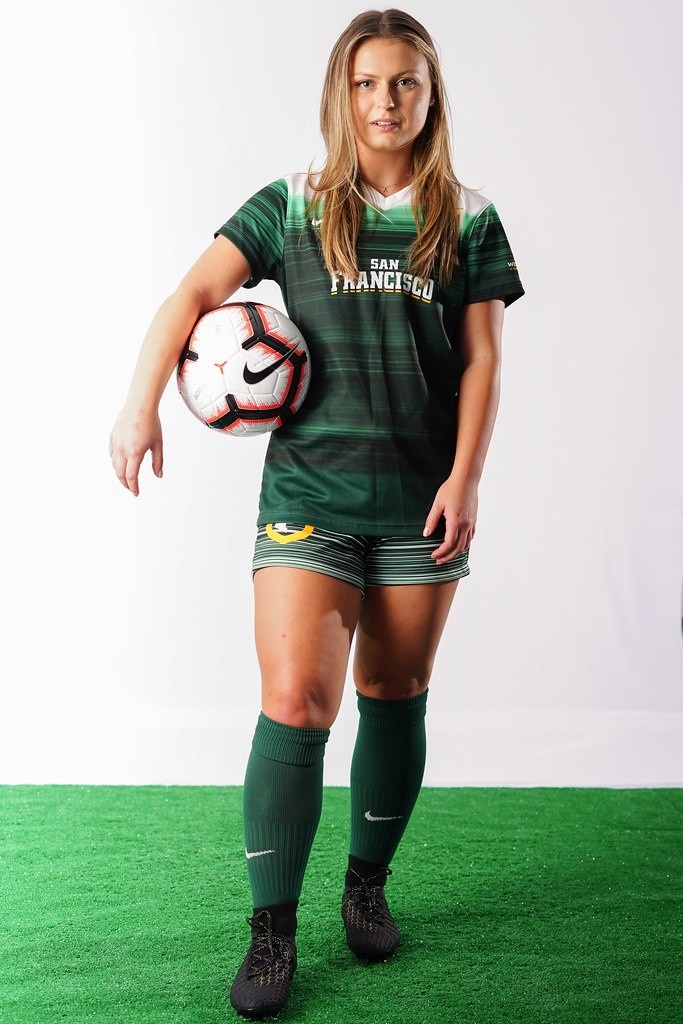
left=176, top=301, right=311, bottom=438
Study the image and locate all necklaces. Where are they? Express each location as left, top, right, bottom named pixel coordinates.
left=364, top=178, right=409, bottom=193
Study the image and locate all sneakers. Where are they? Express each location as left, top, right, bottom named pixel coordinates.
left=230, top=898, right=298, bottom=1016
left=342, top=854, right=399, bottom=959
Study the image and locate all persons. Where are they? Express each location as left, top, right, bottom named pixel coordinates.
left=109, top=9, right=525, bottom=1018
